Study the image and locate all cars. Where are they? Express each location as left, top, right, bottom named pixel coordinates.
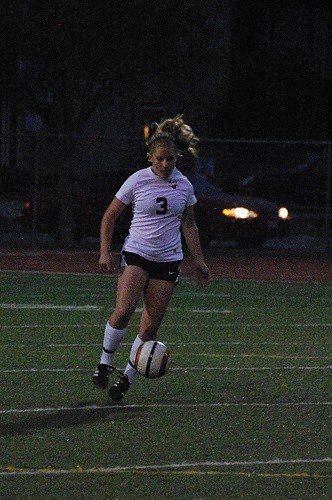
left=20, top=166, right=294, bottom=251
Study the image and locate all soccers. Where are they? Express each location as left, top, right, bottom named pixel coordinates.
left=131, top=338, right=172, bottom=380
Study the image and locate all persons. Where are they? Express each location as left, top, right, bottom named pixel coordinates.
left=92, top=118, right=210, bottom=403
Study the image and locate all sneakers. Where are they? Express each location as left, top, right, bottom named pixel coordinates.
left=93, top=363, right=112, bottom=389
left=108, top=375, right=131, bottom=401
